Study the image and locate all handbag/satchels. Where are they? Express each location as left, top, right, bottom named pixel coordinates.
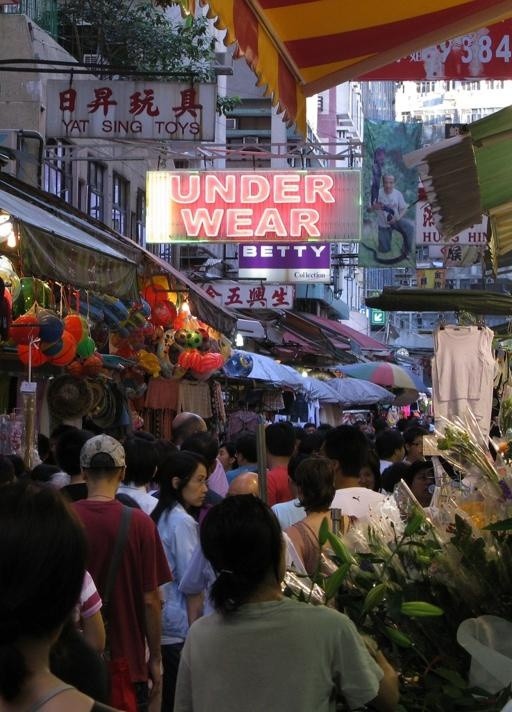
left=104, top=644, right=138, bottom=712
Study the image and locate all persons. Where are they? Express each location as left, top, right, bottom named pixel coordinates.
left=368, top=172, right=415, bottom=261
left=1, top=410, right=439, bottom=712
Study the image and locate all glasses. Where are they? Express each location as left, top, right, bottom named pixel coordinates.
left=168, top=475, right=209, bottom=485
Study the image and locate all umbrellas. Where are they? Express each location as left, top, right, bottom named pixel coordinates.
left=338, top=363, right=433, bottom=399
left=326, top=378, right=396, bottom=407
left=232, top=349, right=303, bottom=388
left=281, top=377, right=343, bottom=405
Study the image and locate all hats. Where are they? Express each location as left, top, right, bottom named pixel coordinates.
left=81, top=432, right=125, bottom=469
left=46, top=375, right=123, bottom=428
